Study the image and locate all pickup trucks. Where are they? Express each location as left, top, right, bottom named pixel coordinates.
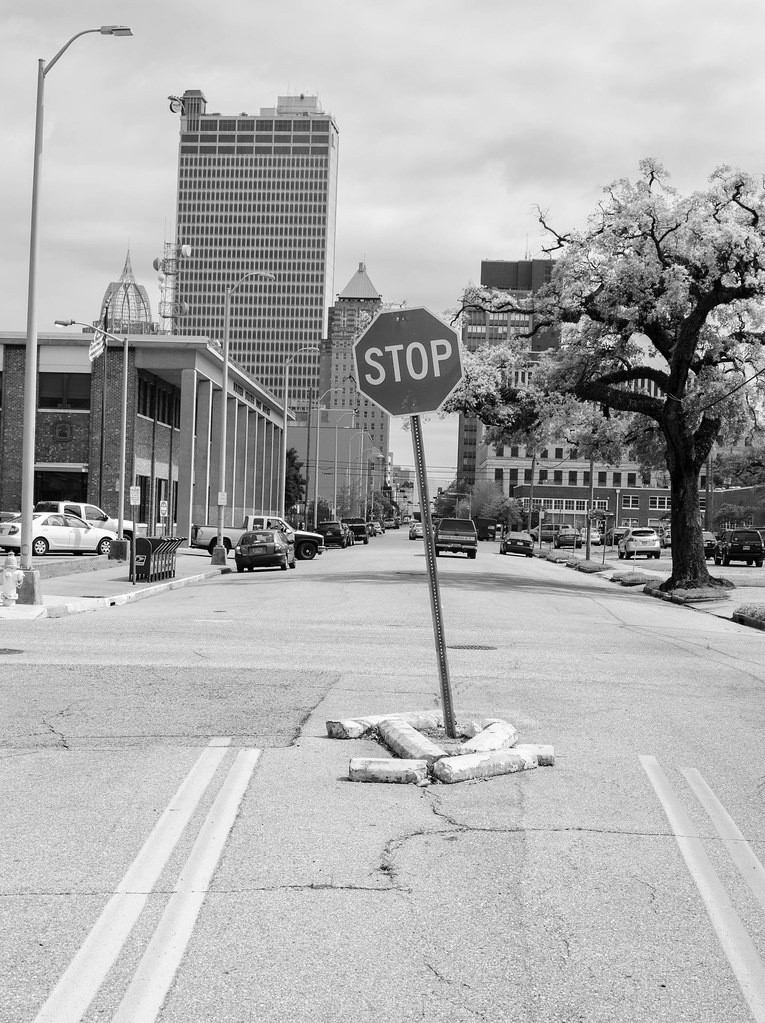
left=189, top=514, right=329, bottom=560
left=340, top=517, right=371, bottom=544
left=0, top=499, right=139, bottom=542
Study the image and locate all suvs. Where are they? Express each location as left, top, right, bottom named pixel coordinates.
left=314, top=521, right=348, bottom=548
left=432, top=517, right=479, bottom=559
left=713, top=529, right=765, bottom=567
left=520, top=523, right=573, bottom=543
left=617, top=526, right=661, bottom=560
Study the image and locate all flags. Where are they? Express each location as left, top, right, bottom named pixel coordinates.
left=89, top=318, right=105, bottom=362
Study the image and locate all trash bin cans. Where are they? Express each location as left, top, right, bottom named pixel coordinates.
left=129, top=536, right=187, bottom=582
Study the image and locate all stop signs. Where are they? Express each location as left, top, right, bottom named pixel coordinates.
left=352, top=306, right=465, bottom=418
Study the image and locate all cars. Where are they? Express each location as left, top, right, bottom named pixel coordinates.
left=659, top=528, right=672, bottom=549
left=580, top=527, right=601, bottom=546
left=364, top=516, right=420, bottom=537
left=234, top=529, right=296, bottom=573
left=553, top=528, right=583, bottom=550
left=0, top=510, right=118, bottom=557
left=702, top=530, right=719, bottom=560
left=409, top=523, right=424, bottom=540
left=342, top=522, right=355, bottom=546
left=598, top=526, right=631, bottom=546
left=500, top=531, right=535, bottom=559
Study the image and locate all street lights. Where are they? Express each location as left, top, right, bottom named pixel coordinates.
left=216, top=270, right=275, bottom=548
left=347, top=432, right=367, bottom=519
left=55, top=320, right=128, bottom=540
left=315, top=387, right=344, bottom=530
left=279, top=347, right=320, bottom=521
left=364, top=452, right=385, bottom=523
